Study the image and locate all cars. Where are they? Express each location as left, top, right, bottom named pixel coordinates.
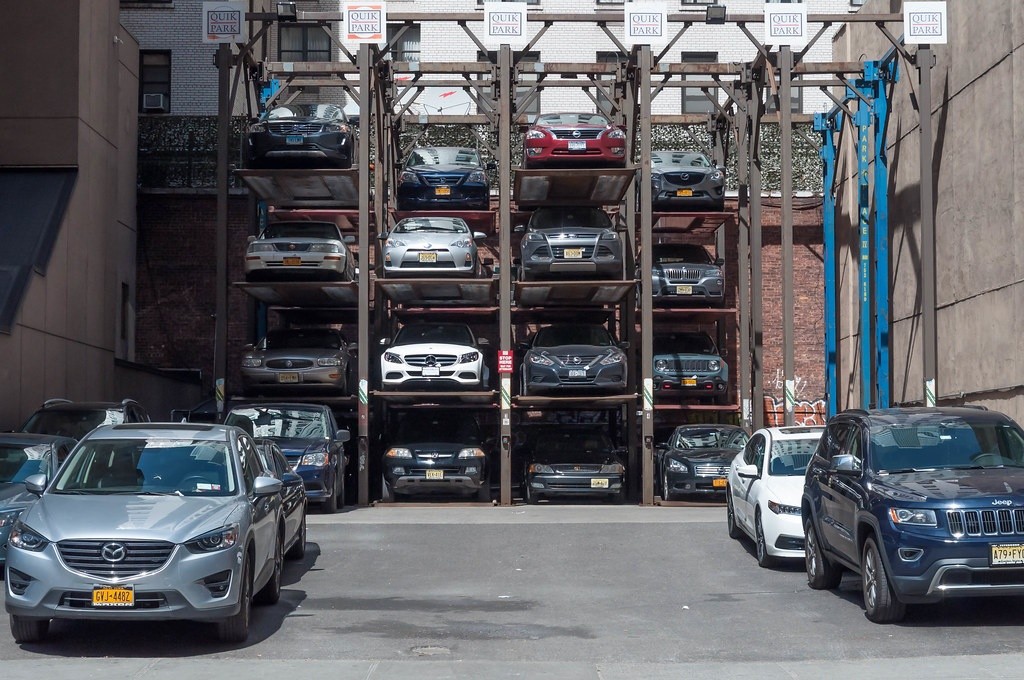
left=249, top=102, right=359, bottom=169
left=652, top=423, right=753, bottom=501
left=4, top=421, right=285, bottom=645
left=514, top=206, right=623, bottom=286
left=518, top=111, right=628, bottom=169
left=252, top=437, right=307, bottom=562
left=633, top=242, right=726, bottom=311
left=518, top=322, right=630, bottom=397
left=378, top=319, right=490, bottom=392
left=239, top=325, right=358, bottom=398
left=0, top=433, right=79, bottom=566
left=725, top=424, right=826, bottom=570
left=634, top=150, right=727, bottom=212
left=518, top=425, right=629, bottom=505
left=376, top=216, right=488, bottom=279
left=392, top=145, right=497, bottom=212
left=243, top=220, right=356, bottom=282
left=636, top=330, right=730, bottom=404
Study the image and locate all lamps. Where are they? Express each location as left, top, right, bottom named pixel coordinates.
left=277, top=2, right=297, bottom=23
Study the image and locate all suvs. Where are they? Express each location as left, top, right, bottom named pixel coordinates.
left=378, top=410, right=497, bottom=503
left=16, top=398, right=152, bottom=464
left=220, top=401, right=351, bottom=514
left=801, top=404, right=1024, bottom=625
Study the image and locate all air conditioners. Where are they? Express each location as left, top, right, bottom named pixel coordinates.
left=143, top=93, right=169, bottom=112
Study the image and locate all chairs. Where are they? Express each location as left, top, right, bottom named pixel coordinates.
left=773, top=456, right=794, bottom=471
left=96, top=449, right=145, bottom=488
left=182, top=440, right=227, bottom=486
